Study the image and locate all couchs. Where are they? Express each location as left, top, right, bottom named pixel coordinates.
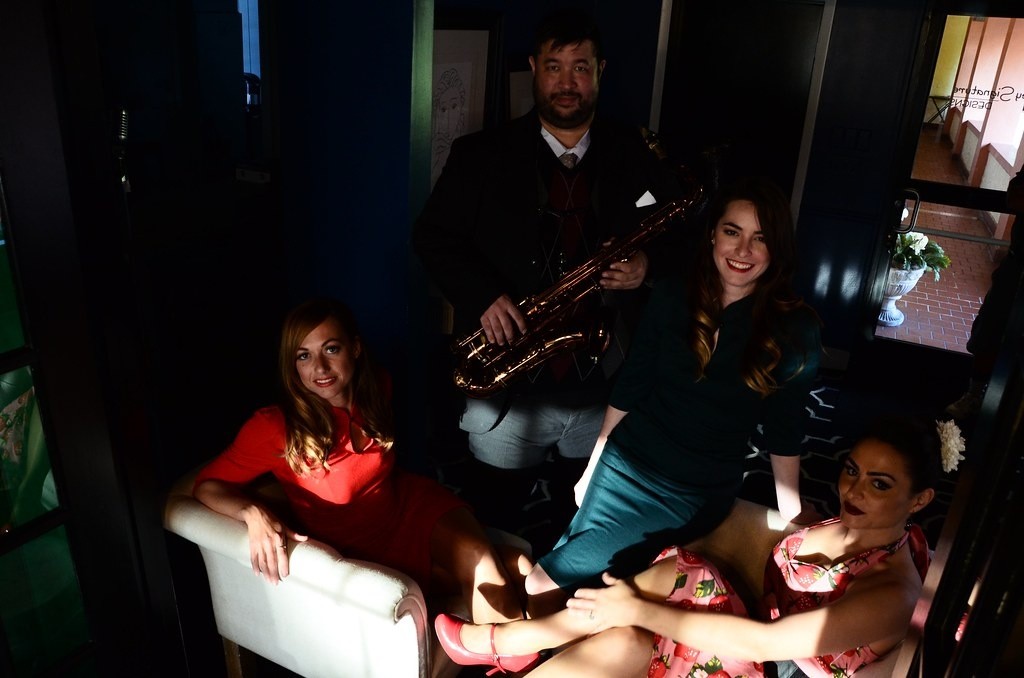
left=166, top=466, right=533, bottom=678
left=557, top=479, right=931, bottom=677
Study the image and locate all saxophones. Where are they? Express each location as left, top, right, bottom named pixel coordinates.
left=439, top=122, right=720, bottom=406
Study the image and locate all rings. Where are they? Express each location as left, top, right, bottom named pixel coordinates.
left=589, top=610, right=595, bottom=620
left=277, top=545, right=287, bottom=549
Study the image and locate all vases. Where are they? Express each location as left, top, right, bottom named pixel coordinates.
left=877, top=262, right=928, bottom=327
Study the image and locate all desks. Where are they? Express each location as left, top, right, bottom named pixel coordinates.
left=926, top=95, right=950, bottom=123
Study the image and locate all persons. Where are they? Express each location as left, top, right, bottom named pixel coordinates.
left=504, top=185, right=825, bottom=656
left=191, top=295, right=532, bottom=626
left=412, top=12, right=689, bottom=554
left=434, top=411, right=966, bottom=678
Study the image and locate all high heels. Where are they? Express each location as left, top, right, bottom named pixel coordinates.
left=434, top=613, right=540, bottom=677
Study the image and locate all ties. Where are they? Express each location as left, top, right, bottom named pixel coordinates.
left=559, top=152, right=578, bottom=168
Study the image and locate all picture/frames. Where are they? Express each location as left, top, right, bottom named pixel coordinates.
left=431, top=10, right=507, bottom=196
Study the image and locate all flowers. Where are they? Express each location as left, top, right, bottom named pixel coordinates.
left=892, top=232, right=951, bottom=282
left=936, top=420, right=967, bottom=474
